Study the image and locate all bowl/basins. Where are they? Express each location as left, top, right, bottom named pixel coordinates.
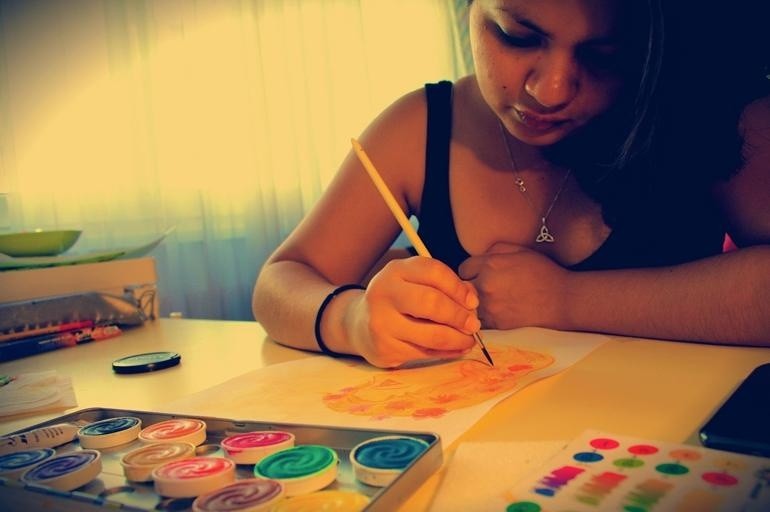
left=0, top=230, right=82, bottom=258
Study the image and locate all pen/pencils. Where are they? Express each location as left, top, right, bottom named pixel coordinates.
left=1, top=320, right=120, bottom=343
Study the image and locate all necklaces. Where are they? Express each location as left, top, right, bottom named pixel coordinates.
left=497, top=117, right=568, bottom=244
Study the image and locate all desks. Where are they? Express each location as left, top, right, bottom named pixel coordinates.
left=1, top=317, right=770, bottom=512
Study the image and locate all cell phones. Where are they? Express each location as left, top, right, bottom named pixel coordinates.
left=699, top=362, right=770, bottom=456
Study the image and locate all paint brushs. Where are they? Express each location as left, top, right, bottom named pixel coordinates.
left=353, top=142, right=495, bottom=366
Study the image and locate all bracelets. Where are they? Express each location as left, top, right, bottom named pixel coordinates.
left=314, top=282, right=368, bottom=359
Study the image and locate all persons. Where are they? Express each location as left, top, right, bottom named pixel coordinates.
left=250, top=1, right=769, bottom=370
